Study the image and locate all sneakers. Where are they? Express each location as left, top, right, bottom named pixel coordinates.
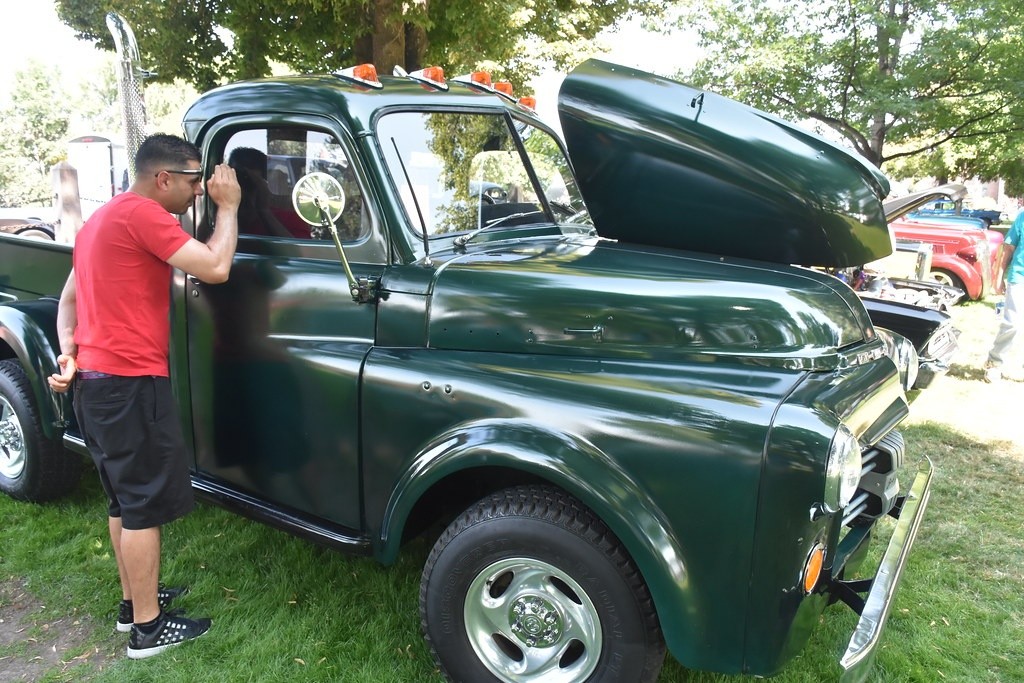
left=126, top=608, right=214, bottom=660
left=117, top=582, right=187, bottom=632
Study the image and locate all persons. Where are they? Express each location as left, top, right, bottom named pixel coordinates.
left=982, top=207, right=1024, bottom=383
left=226, top=145, right=313, bottom=238
left=48, top=134, right=242, bottom=661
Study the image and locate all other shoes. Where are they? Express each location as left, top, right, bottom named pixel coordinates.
left=984, top=358, right=1002, bottom=383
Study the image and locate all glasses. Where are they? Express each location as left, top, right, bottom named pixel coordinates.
left=155, top=164, right=203, bottom=182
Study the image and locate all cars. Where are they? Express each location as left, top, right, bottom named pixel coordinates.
left=568, top=182, right=1014, bottom=388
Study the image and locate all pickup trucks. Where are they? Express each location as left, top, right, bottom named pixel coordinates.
left=1, top=8, right=937, bottom=683
